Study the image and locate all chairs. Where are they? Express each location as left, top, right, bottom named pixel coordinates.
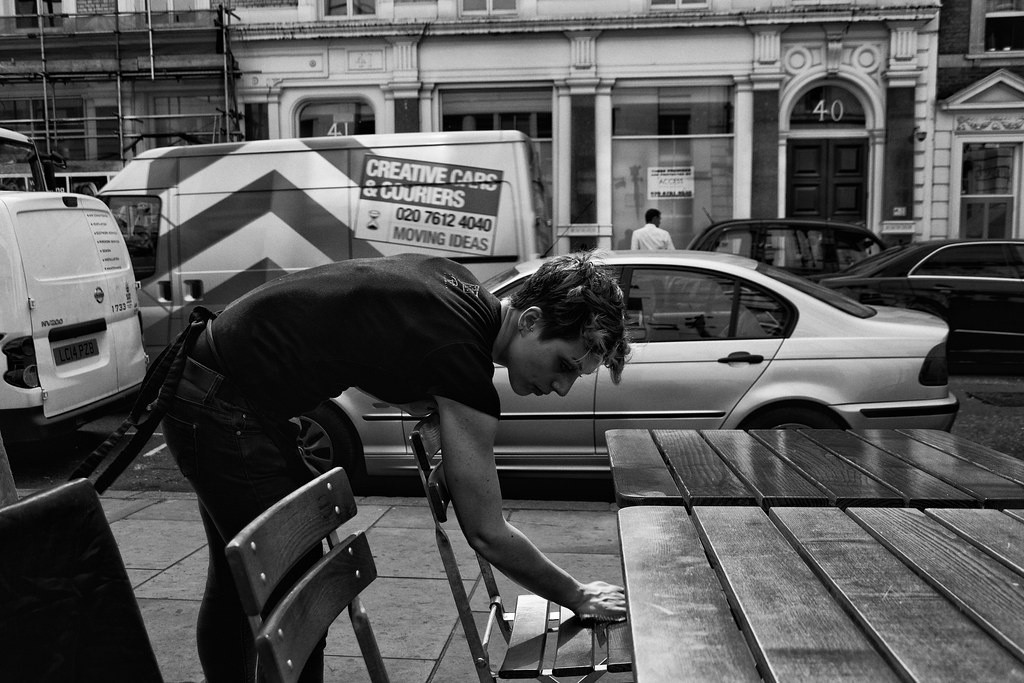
left=407, top=414, right=634, bottom=683
left=0, top=476, right=164, bottom=683
left=225, top=467, right=389, bottom=683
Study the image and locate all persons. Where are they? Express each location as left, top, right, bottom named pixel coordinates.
left=679, top=280, right=768, bottom=338
left=631, top=208, right=675, bottom=251
left=161, top=256, right=631, bottom=683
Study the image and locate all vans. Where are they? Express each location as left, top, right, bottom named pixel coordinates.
left=0, top=129, right=551, bottom=362
left=0, top=189, right=152, bottom=444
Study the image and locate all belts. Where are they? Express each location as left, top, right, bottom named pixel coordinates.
left=184, top=358, right=249, bottom=409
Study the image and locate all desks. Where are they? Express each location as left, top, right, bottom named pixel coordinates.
left=603, top=428, right=1024, bottom=683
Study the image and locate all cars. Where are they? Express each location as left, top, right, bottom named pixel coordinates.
left=290, top=248, right=960, bottom=497
left=818, top=235, right=1024, bottom=367
left=634, top=217, right=890, bottom=314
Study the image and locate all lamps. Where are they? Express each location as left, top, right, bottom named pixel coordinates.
left=908, top=126, right=927, bottom=144
